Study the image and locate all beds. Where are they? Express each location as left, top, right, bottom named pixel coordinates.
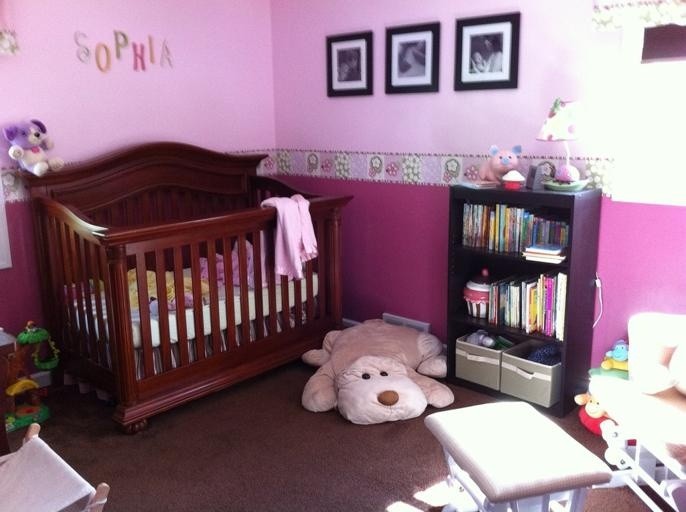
left=11, top=139, right=355, bottom=438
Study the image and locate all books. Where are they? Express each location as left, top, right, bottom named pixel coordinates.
left=461, top=204, right=573, bottom=341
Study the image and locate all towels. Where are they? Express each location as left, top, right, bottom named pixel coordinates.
left=258, top=192, right=321, bottom=284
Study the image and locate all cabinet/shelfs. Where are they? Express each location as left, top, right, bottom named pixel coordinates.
left=445, top=183, right=604, bottom=419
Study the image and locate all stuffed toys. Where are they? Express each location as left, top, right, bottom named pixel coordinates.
left=601, top=341, right=628, bottom=377
left=478, top=145, right=522, bottom=182
left=574, top=390, right=611, bottom=419
left=600, top=421, right=633, bottom=469
left=300, top=320, right=454, bottom=424
left=1, top=120, right=65, bottom=178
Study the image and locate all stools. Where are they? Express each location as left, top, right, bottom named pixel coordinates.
left=0, top=421, right=112, bottom=512
left=424, top=400, right=613, bottom=512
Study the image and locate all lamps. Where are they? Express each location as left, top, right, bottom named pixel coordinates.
left=536, top=97, right=596, bottom=192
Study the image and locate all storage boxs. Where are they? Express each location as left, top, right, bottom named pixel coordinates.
left=500, top=338, right=562, bottom=410
left=454, top=332, right=527, bottom=391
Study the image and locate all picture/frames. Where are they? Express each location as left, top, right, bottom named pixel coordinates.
left=453, top=10, right=524, bottom=93
left=325, top=29, right=374, bottom=98
left=383, top=20, right=442, bottom=95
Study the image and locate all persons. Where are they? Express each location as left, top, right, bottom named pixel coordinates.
left=403, top=41, right=426, bottom=77
left=473, top=36, right=503, bottom=72
